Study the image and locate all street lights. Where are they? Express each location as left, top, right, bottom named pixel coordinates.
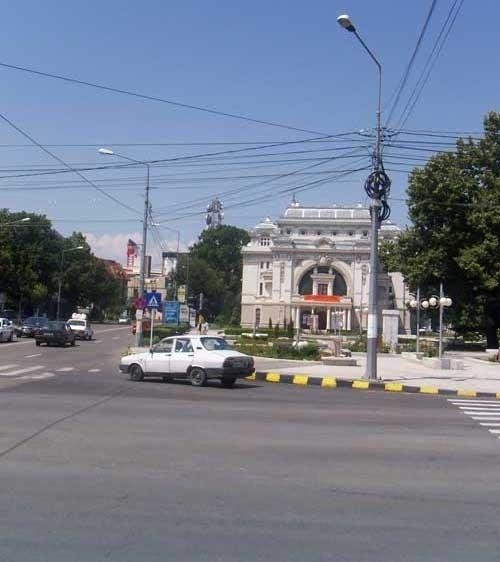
left=56, top=246, right=84, bottom=321
left=96, top=146, right=153, bottom=348
left=429, top=282, right=453, bottom=359
left=408, top=286, right=429, bottom=358
left=153, top=222, right=181, bottom=300
left=335, top=14, right=389, bottom=380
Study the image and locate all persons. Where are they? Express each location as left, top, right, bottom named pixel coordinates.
left=195, top=316, right=209, bottom=334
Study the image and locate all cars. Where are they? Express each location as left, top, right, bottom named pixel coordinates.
left=64, top=318, right=94, bottom=341
left=132, top=318, right=152, bottom=335
left=34, top=320, right=77, bottom=348
left=0, top=317, right=15, bottom=343
left=118, top=315, right=132, bottom=324
left=119, top=332, right=256, bottom=389
left=15, top=315, right=48, bottom=337
